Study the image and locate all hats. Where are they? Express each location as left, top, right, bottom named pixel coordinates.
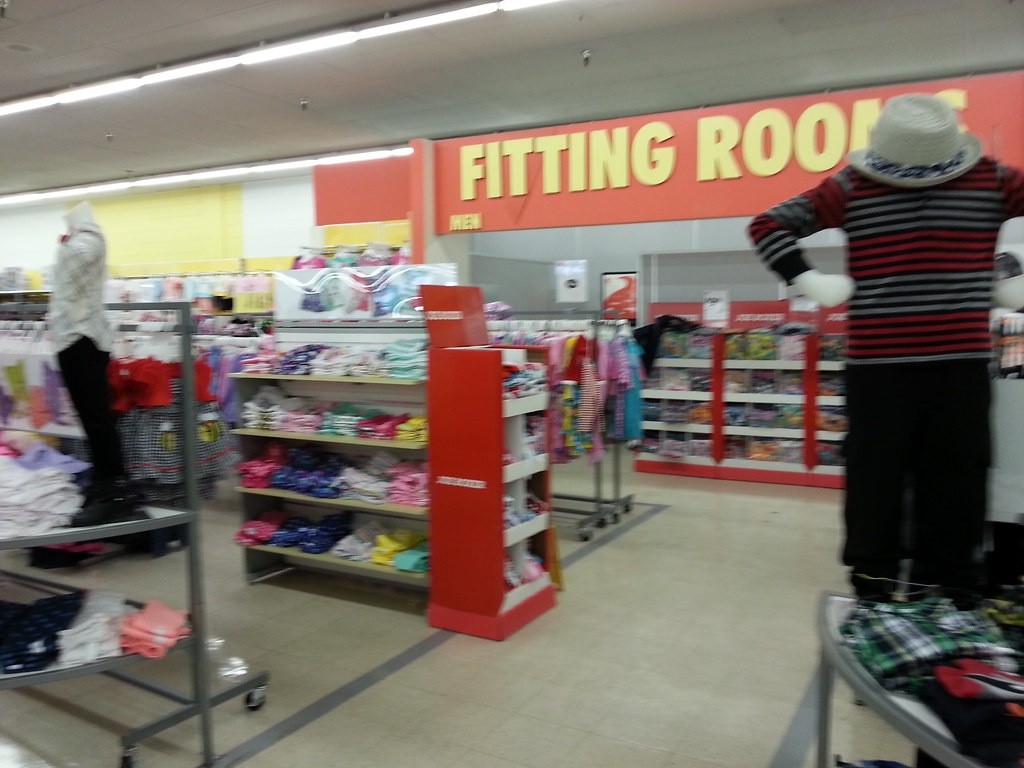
left=851, top=93, right=981, bottom=187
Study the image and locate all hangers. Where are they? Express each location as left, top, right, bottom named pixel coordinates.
left=332, top=245, right=359, bottom=257
left=298, top=247, right=324, bottom=260
left=0, top=315, right=273, bottom=359
left=361, top=242, right=392, bottom=258
left=485, top=318, right=632, bottom=338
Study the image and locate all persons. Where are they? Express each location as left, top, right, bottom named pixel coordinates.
left=48, top=200, right=139, bottom=523
left=745, top=96, right=1024, bottom=598
left=293, top=246, right=412, bottom=268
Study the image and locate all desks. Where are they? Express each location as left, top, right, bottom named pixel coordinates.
left=819, top=587, right=978, bottom=768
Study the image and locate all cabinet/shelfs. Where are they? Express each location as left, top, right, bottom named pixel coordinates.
left=227, top=373, right=428, bottom=589
left=427, top=345, right=555, bottom=642
left=634, top=334, right=846, bottom=489
left=1, top=300, right=271, bottom=767
